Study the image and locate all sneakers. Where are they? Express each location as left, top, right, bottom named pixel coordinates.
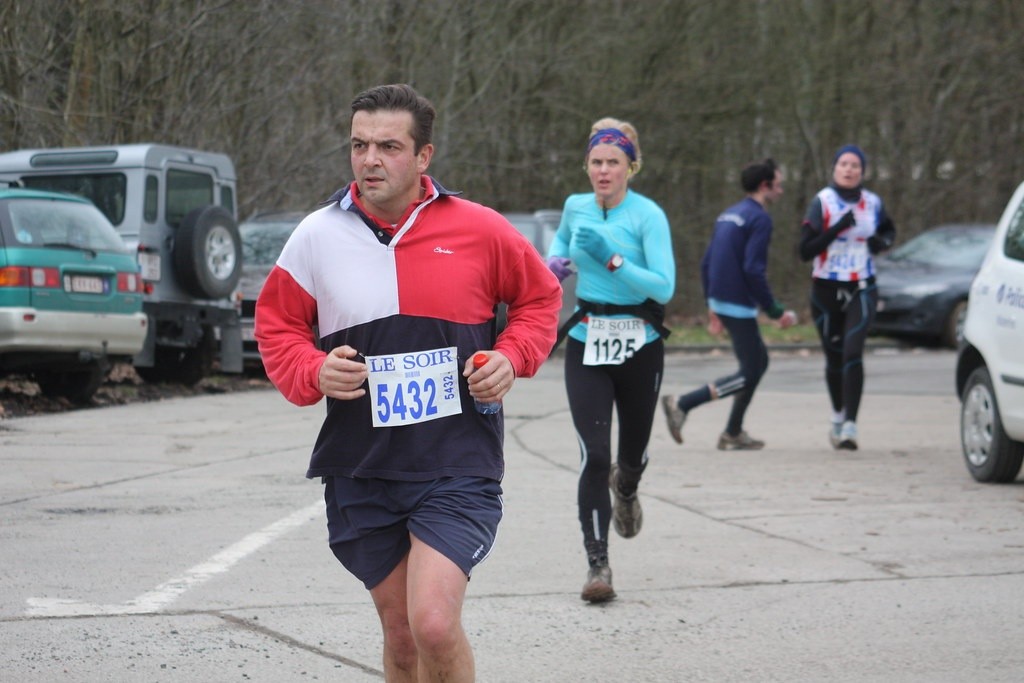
left=581, top=566, right=617, bottom=602
left=718, top=430, right=765, bottom=450
left=662, top=394, right=686, bottom=444
left=609, top=461, right=643, bottom=538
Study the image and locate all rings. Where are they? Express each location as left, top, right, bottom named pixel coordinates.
left=497, top=383, right=502, bottom=391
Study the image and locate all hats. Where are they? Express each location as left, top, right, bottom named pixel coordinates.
left=834, top=146, right=865, bottom=171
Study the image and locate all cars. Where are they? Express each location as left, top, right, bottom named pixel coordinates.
left=236, top=214, right=319, bottom=384
left=953, top=181, right=1024, bottom=484
left=0, top=177, right=150, bottom=399
left=863, top=220, right=1002, bottom=350
left=487, top=209, right=581, bottom=352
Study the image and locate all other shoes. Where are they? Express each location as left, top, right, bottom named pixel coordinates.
left=830, top=421, right=842, bottom=448
left=838, top=420, right=860, bottom=450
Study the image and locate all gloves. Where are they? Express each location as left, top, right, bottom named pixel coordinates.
left=548, top=255, right=574, bottom=283
left=868, top=234, right=885, bottom=255
left=833, top=211, right=856, bottom=232
left=574, top=226, right=615, bottom=267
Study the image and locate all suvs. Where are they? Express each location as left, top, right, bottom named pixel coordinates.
left=0, top=142, right=244, bottom=386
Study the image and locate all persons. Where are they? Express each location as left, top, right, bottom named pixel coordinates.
left=796, top=148, right=897, bottom=450
left=548, top=118, right=675, bottom=599
left=254, top=84, right=563, bottom=683
left=662, top=160, right=794, bottom=452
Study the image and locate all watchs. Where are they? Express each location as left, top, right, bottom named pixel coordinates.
left=608, top=254, right=623, bottom=271
